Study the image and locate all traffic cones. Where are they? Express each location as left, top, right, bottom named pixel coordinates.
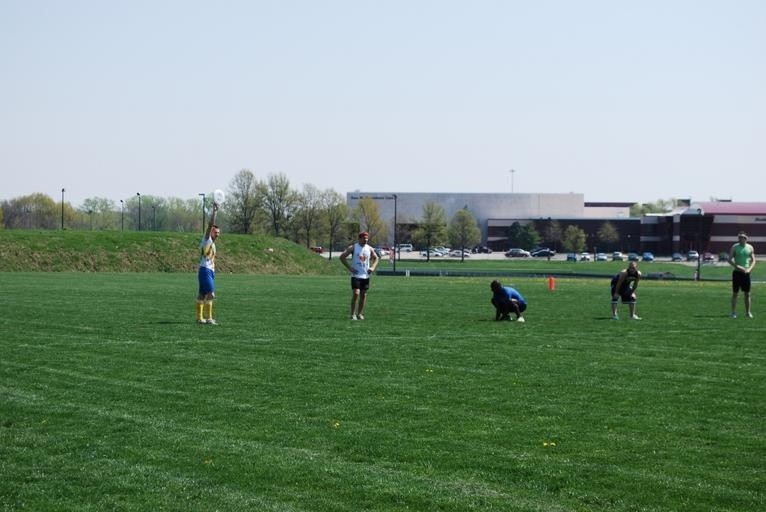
left=548, top=276, right=556, bottom=291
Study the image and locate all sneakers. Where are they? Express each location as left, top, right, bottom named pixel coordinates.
left=612, top=315, right=619, bottom=320
left=730, top=312, right=736, bottom=318
left=744, top=312, right=753, bottom=319
left=351, top=313, right=365, bottom=320
left=499, top=315, right=525, bottom=323
left=197, top=319, right=217, bottom=325
left=629, top=314, right=642, bottom=320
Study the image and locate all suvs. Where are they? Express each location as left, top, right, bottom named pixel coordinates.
left=309, top=247, right=322, bottom=254
left=686, top=250, right=699, bottom=260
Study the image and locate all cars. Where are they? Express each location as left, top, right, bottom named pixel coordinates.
left=642, top=252, right=653, bottom=260
left=672, top=252, right=682, bottom=261
left=580, top=252, right=589, bottom=260
left=718, top=251, right=729, bottom=260
left=596, top=252, right=607, bottom=261
left=627, top=252, right=638, bottom=261
left=702, top=252, right=714, bottom=260
left=612, top=252, right=623, bottom=260
left=376, top=243, right=557, bottom=259
left=566, top=253, right=577, bottom=261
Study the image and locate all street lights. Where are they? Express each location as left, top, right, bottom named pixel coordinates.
left=120, top=199, right=124, bottom=233
left=136, top=192, right=140, bottom=231
left=695, top=207, right=704, bottom=281
left=60, top=187, right=65, bottom=229
left=197, top=193, right=204, bottom=233
left=391, top=193, right=396, bottom=271
left=151, top=205, right=155, bottom=231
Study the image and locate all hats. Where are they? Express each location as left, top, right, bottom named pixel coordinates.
left=358, top=232, right=368, bottom=239
left=738, top=231, right=748, bottom=242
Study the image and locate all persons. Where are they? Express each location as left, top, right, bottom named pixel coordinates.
left=196, top=202, right=222, bottom=327
left=490, top=280, right=529, bottom=323
left=609, top=261, right=644, bottom=321
left=339, top=231, right=380, bottom=321
left=725, top=229, right=758, bottom=320
